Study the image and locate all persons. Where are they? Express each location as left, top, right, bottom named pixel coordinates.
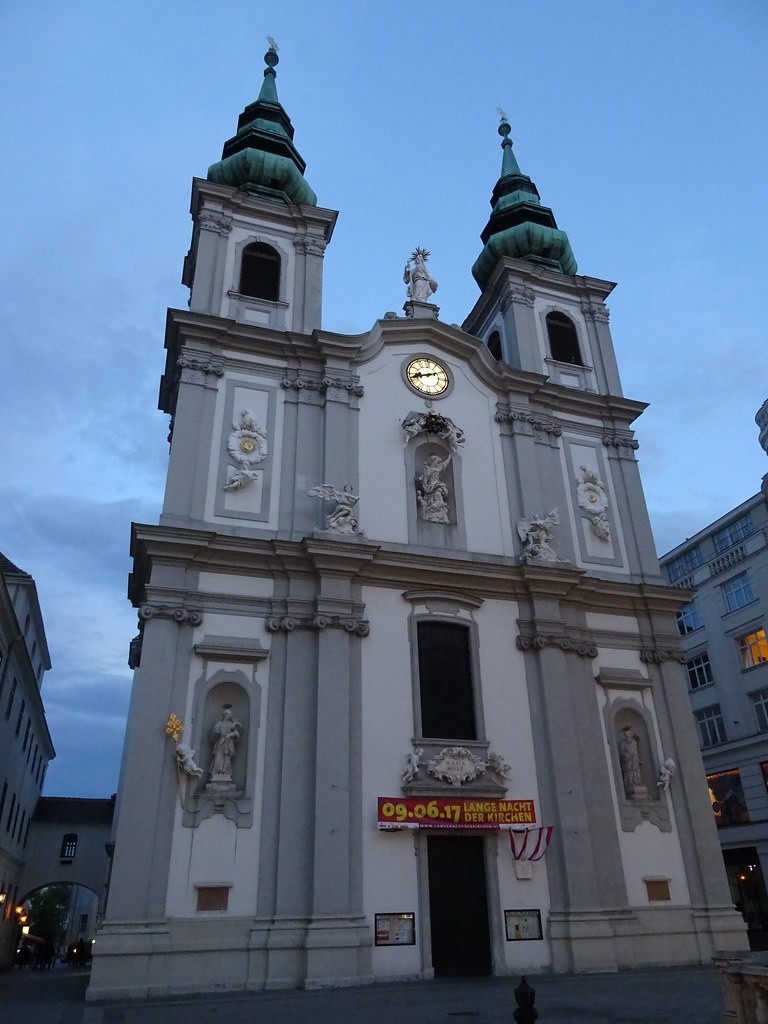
left=581, top=463, right=604, bottom=488
left=438, top=421, right=465, bottom=454
left=417, top=452, right=454, bottom=515
left=592, top=513, right=612, bottom=542
left=484, top=751, right=511, bottom=781
left=404, top=416, right=427, bottom=443
left=517, top=507, right=559, bottom=550
left=306, top=481, right=360, bottom=534
left=224, top=461, right=258, bottom=491
left=400, top=746, right=426, bottom=781
left=239, top=408, right=258, bottom=432
left=175, top=705, right=241, bottom=778
left=14, top=938, right=92, bottom=971
left=713, top=779, right=748, bottom=827
left=619, top=726, right=676, bottom=791
left=404, top=246, right=438, bottom=303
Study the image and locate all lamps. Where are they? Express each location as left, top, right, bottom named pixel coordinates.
left=13, top=903, right=24, bottom=915
left=7, top=913, right=27, bottom=923
left=0, top=890, right=7, bottom=904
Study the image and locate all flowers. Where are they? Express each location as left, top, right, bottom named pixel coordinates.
left=165, top=714, right=184, bottom=734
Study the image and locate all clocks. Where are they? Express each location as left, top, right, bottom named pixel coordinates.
left=406, top=358, right=450, bottom=396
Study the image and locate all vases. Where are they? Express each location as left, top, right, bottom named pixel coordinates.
left=171, top=731, right=179, bottom=742
